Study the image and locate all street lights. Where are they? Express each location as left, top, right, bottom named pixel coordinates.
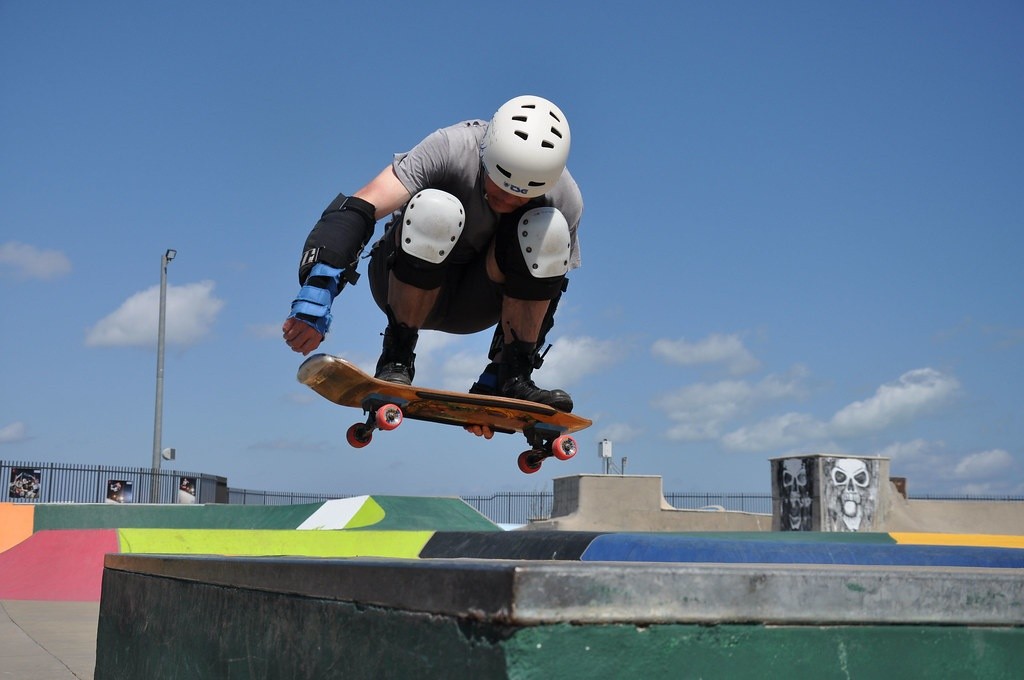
left=149, top=249, right=177, bottom=503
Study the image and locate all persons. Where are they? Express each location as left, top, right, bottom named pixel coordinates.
left=282, top=96, right=583, bottom=414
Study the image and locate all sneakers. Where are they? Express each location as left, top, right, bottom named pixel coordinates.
left=374, top=304, right=419, bottom=385
left=468, top=321, right=573, bottom=413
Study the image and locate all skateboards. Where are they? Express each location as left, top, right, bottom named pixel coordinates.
left=296, top=353, right=593, bottom=477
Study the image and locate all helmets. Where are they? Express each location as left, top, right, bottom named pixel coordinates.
left=480, top=95, right=571, bottom=198
